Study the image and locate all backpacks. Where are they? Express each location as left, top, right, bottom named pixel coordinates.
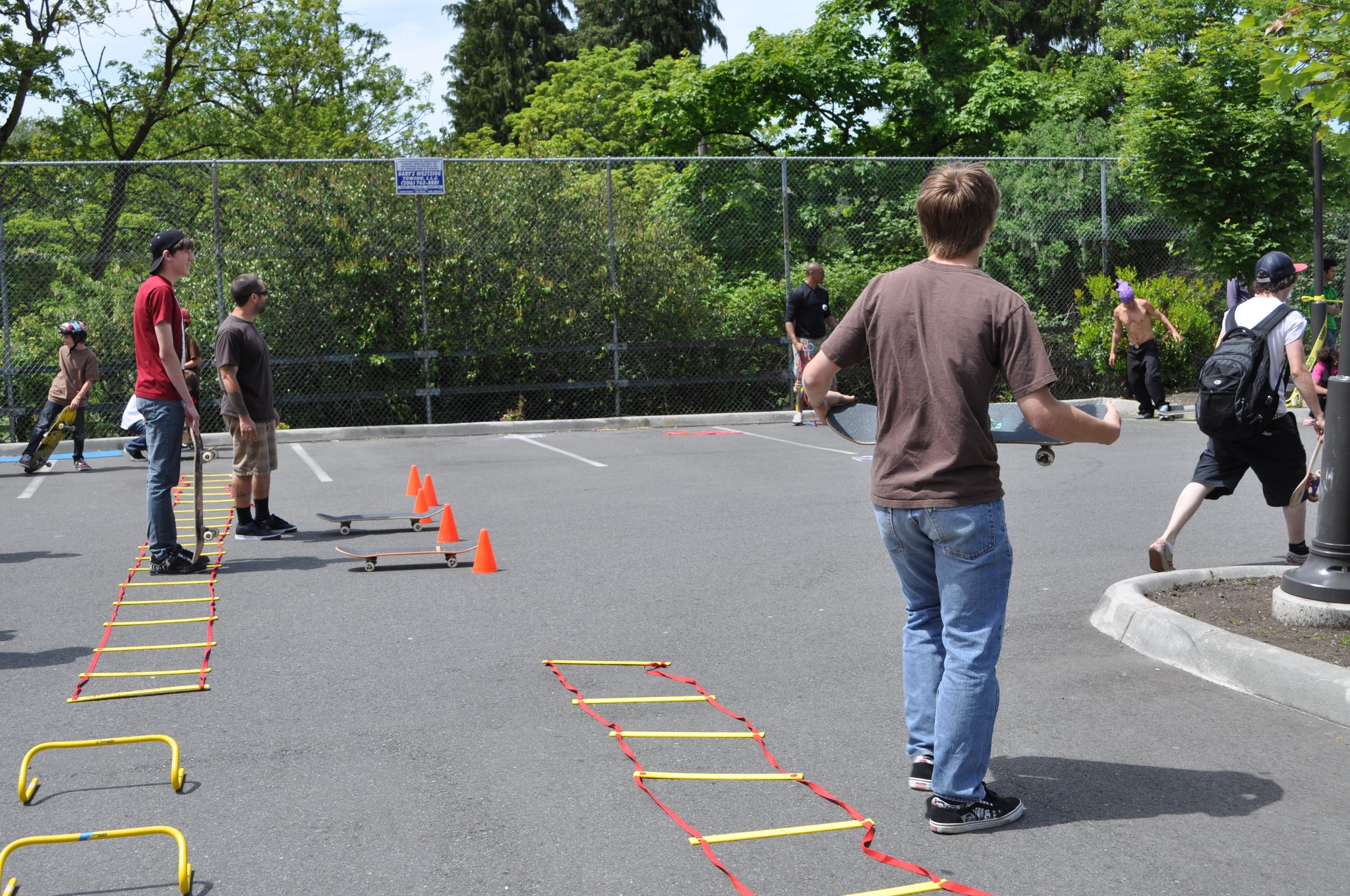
left=1195, top=302, right=1296, bottom=440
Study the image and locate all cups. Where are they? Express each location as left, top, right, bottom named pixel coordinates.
left=1291, top=396, right=1298, bottom=406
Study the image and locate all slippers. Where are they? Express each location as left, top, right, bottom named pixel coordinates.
left=1303, top=419, right=1315, bottom=425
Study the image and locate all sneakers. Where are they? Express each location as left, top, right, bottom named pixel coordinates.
left=179, top=547, right=210, bottom=564
left=74, top=458, right=91, bottom=470
left=1285, top=546, right=1310, bottom=566
left=1158, top=414, right=1169, bottom=420
left=149, top=552, right=208, bottom=575
left=792, top=411, right=803, bottom=424
left=908, top=755, right=934, bottom=791
left=18, top=454, right=32, bottom=468
left=929, top=781, right=1024, bottom=833
left=181, top=445, right=190, bottom=451
left=1149, top=538, right=1176, bottom=572
left=1136, top=410, right=1155, bottom=419
left=235, top=519, right=281, bottom=540
left=123, top=444, right=148, bottom=461
left=254, top=514, right=297, bottom=533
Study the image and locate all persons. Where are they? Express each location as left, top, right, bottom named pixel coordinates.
left=121, top=391, right=190, bottom=461
left=180, top=307, right=203, bottom=451
left=1303, top=345, right=1338, bottom=426
left=785, top=262, right=840, bottom=425
left=1109, top=278, right=1183, bottom=421
left=18, top=320, right=99, bottom=473
left=1147, top=251, right=1324, bottom=570
left=133, top=230, right=210, bottom=576
left=802, top=163, right=1118, bottom=835
left=1309, top=258, right=1340, bottom=351
left=216, top=274, right=296, bottom=541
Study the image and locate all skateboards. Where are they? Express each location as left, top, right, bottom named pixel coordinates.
left=24, top=405, right=76, bottom=473
left=827, top=402, right=1109, bottom=466
left=189, top=416, right=219, bottom=565
left=1157, top=405, right=1196, bottom=421
left=1289, top=433, right=1323, bottom=508
left=316, top=504, right=443, bottom=535
left=335, top=538, right=477, bottom=572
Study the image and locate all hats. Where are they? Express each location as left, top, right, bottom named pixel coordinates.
left=1255, top=251, right=1308, bottom=282
left=1116, top=279, right=1134, bottom=303
left=149, top=229, right=186, bottom=275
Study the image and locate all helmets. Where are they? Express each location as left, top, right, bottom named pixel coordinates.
left=58, top=320, right=86, bottom=335
left=181, top=308, right=191, bottom=325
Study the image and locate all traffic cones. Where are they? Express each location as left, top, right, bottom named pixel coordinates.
left=472, top=529, right=497, bottom=573
left=406, top=464, right=422, bottom=496
left=423, top=474, right=438, bottom=507
left=437, top=503, right=460, bottom=543
left=413, top=489, right=432, bottom=524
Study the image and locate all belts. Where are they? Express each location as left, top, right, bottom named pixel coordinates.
left=1129, top=338, right=1155, bottom=348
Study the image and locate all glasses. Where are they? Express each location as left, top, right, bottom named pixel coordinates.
left=255, top=290, right=268, bottom=297
left=813, top=271, right=825, bottom=275
